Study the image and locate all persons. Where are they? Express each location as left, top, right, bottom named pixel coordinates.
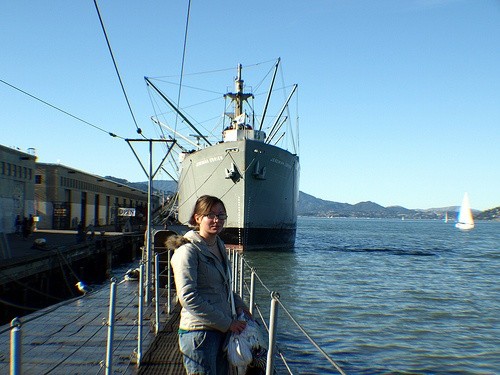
left=78, top=221, right=94, bottom=241
left=15, top=214, right=35, bottom=241
left=164, top=194, right=255, bottom=375
left=246, top=124, right=252, bottom=129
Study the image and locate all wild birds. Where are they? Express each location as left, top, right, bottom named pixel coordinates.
left=75, top=281, right=93, bottom=297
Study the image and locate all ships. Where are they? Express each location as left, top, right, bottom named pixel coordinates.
left=143, top=58, right=300, bottom=251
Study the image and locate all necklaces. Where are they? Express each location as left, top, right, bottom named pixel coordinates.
left=207, top=240, right=217, bottom=247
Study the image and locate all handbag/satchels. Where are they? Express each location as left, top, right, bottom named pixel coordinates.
left=225, top=310, right=267, bottom=366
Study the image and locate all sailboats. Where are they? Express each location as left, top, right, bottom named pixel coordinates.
left=456, top=192, right=475, bottom=230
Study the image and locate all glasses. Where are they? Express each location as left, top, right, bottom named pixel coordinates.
left=203, top=213, right=228, bottom=220
